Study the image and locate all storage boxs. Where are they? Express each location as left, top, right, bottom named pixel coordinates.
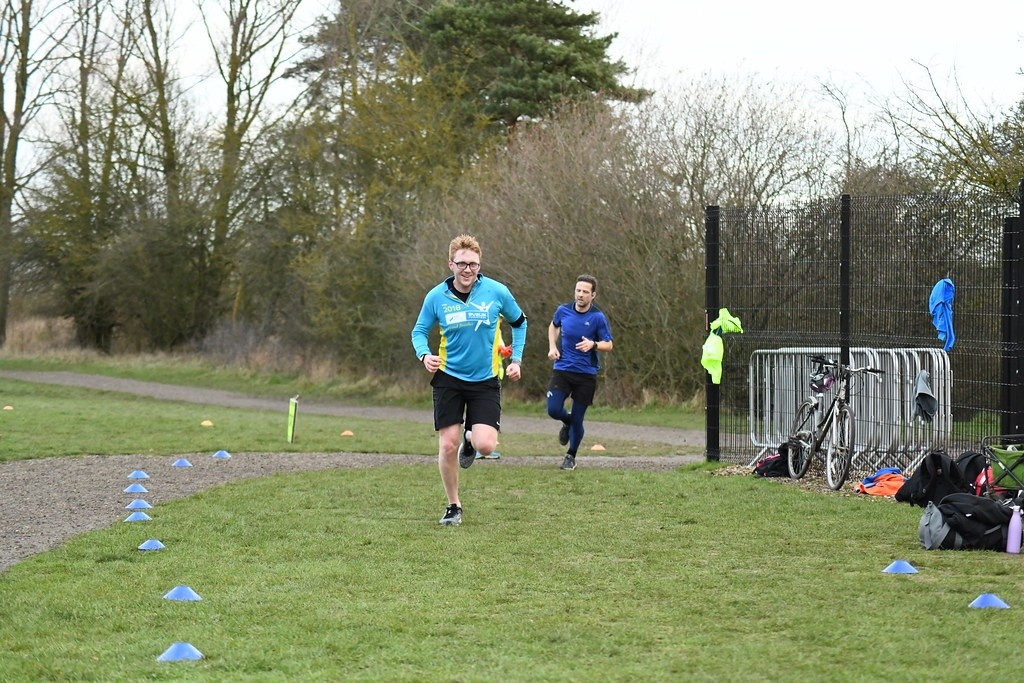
left=981, top=433, right=1024, bottom=503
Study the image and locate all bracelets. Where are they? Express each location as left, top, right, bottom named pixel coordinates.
left=421, top=354, right=426, bottom=363
left=592, top=341, right=597, bottom=350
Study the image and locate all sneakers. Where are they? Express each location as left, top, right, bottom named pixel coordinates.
left=438, top=503, right=462, bottom=526
left=558, top=412, right=572, bottom=446
left=560, top=453, right=577, bottom=470
left=474, top=450, right=501, bottom=460
left=458, top=428, right=477, bottom=469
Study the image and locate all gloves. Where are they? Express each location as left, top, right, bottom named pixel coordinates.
left=498, top=344, right=512, bottom=358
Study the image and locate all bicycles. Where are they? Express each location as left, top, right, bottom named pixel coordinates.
left=786, top=352, right=887, bottom=491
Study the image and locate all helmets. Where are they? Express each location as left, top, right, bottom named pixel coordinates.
left=809, top=358, right=835, bottom=393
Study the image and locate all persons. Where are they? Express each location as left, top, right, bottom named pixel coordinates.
left=475, top=327, right=513, bottom=459
left=410, top=233, right=528, bottom=528
left=547, top=273, right=613, bottom=471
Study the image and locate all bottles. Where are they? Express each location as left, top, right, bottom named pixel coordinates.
left=1006, top=505, right=1022, bottom=553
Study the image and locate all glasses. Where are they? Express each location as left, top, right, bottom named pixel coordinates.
left=451, top=260, right=481, bottom=271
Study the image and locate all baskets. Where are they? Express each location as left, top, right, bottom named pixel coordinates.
left=987, top=443, right=1024, bottom=489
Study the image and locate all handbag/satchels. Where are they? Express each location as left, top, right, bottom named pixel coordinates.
left=917, top=493, right=1024, bottom=552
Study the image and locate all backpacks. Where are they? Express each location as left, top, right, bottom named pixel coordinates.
left=752, top=442, right=800, bottom=478
left=895, top=450, right=990, bottom=510
